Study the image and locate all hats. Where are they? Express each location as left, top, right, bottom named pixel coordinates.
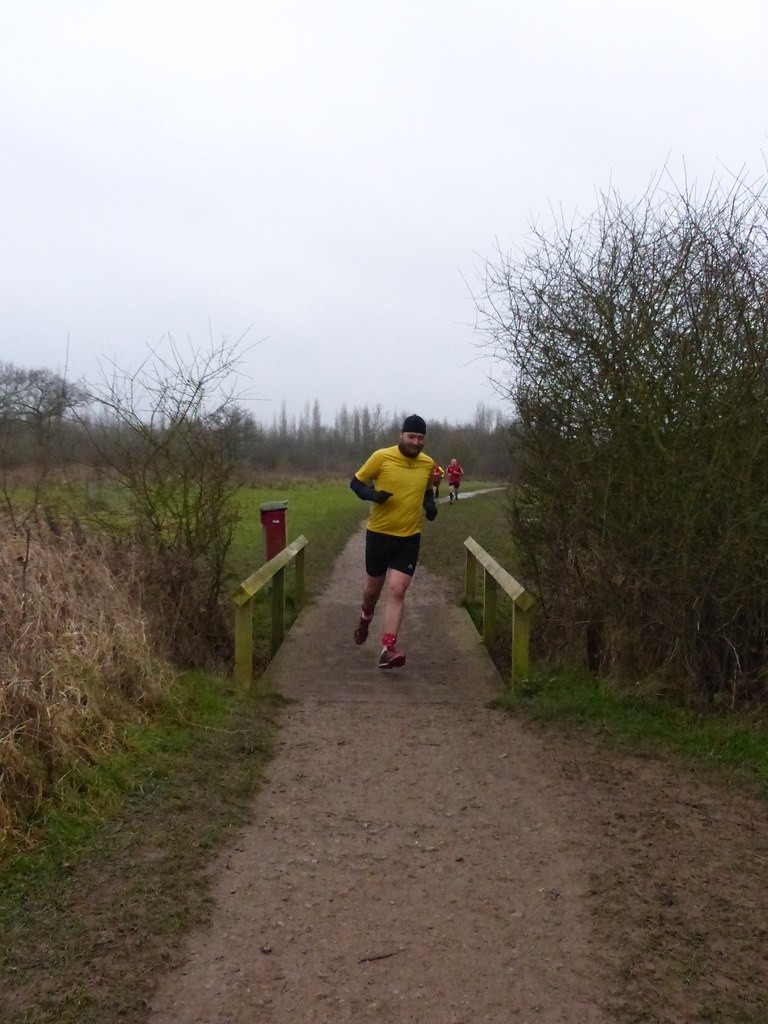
left=403, top=415, right=426, bottom=435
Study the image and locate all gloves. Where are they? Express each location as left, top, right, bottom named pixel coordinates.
left=426, top=506, right=438, bottom=520
left=372, top=489, right=393, bottom=504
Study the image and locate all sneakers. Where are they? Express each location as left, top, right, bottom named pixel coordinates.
left=354, top=615, right=373, bottom=644
left=378, top=646, right=407, bottom=669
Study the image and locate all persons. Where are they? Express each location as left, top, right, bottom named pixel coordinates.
left=432, top=459, right=445, bottom=500
left=446, top=456, right=466, bottom=505
left=349, top=414, right=439, bottom=670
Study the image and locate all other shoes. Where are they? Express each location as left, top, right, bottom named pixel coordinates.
left=455, top=496, right=458, bottom=500
left=450, top=502, right=453, bottom=504
